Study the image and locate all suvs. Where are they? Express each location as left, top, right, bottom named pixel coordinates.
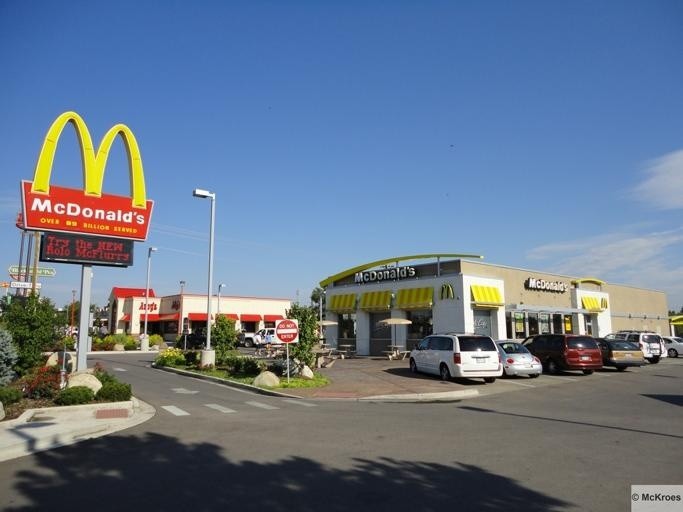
left=238, top=328, right=285, bottom=347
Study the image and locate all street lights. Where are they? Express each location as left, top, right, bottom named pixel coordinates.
left=217, top=283, right=226, bottom=316
left=179, top=280, right=186, bottom=337
left=193, top=187, right=216, bottom=369
left=318, top=291, right=325, bottom=344
left=70, top=290, right=76, bottom=338
left=137, top=247, right=157, bottom=352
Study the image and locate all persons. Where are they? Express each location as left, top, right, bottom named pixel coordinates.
left=233, top=329, right=272, bottom=356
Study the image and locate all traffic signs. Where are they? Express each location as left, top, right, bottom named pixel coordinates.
left=10, top=280, right=42, bottom=290
left=7, top=265, right=56, bottom=277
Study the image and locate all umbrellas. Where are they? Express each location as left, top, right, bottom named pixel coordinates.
left=379, top=318, right=412, bottom=347
left=315, top=320, right=338, bottom=335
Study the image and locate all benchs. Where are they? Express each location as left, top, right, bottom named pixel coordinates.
left=266, top=344, right=412, bottom=368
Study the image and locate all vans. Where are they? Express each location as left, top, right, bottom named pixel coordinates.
left=520, top=332, right=603, bottom=375
left=603, top=330, right=667, bottom=364
left=409, top=332, right=503, bottom=383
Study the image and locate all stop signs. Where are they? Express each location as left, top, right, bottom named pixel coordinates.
left=274, top=319, right=298, bottom=343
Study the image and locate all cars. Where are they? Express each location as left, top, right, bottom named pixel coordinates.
left=593, top=338, right=644, bottom=372
left=493, top=339, right=542, bottom=378
left=53, top=319, right=107, bottom=339
left=661, top=336, right=682, bottom=358
